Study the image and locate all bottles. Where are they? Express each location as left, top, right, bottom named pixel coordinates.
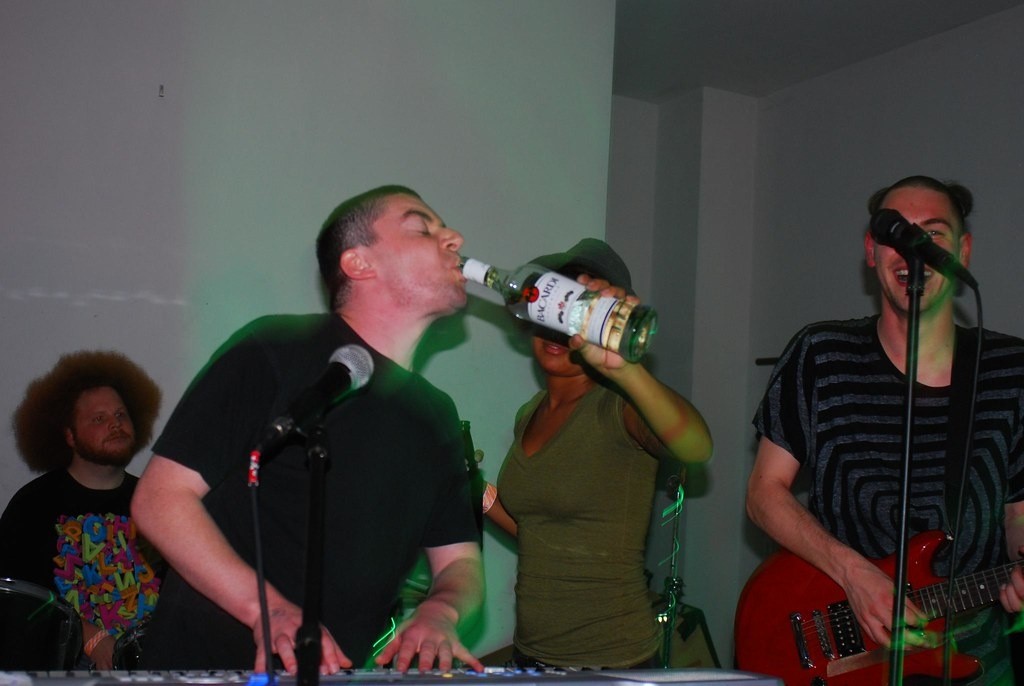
left=456, top=255, right=657, bottom=364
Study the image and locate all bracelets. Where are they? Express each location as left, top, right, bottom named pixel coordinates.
left=85, top=629, right=110, bottom=657
left=482, top=482, right=498, bottom=514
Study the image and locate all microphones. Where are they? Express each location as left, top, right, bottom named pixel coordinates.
left=270, top=344, right=375, bottom=437
left=869, top=207, right=980, bottom=290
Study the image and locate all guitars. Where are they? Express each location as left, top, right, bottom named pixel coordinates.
left=735, top=530, right=1024, bottom=686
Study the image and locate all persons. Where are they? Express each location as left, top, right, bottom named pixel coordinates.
left=0, top=349, right=163, bottom=672
left=475, top=235, right=713, bottom=675
left=747, top=176, right=1024, bottom=686
left=131, top=185, right=486, bottom=676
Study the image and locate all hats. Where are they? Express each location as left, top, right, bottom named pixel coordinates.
left=529, top=238, right=635, bottom=295
